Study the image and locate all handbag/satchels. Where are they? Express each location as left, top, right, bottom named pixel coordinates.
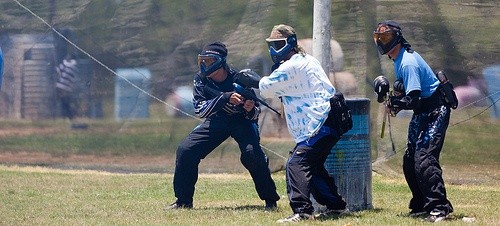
left=329, top=92, right=354, bottom=133
left=436, top=71, right=458, bottom=109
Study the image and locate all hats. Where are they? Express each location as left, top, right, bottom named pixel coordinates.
left=374, top=21, right=402, bottom=36
left=200, top=42, right=228, bottom=59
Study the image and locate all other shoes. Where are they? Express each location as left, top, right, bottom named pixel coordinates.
left=264, top=201, right=277, bottom=210
left=315, top=208, right=352, bottom=220
left=422, top=210, right=449, bottom=222
left=275, top=214, right=315, bottom=223
left=164, top=198, right=194, bottom=210
left=407, top=210, right=429, bottom=217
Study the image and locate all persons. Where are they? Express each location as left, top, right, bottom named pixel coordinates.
left=373, top=20, right=455, bottom=223
left=259, top=24, right=352, bottom=222
left=47, top=52, right=78, bottom=120
left=162, top=42, right=280, bottom=211
